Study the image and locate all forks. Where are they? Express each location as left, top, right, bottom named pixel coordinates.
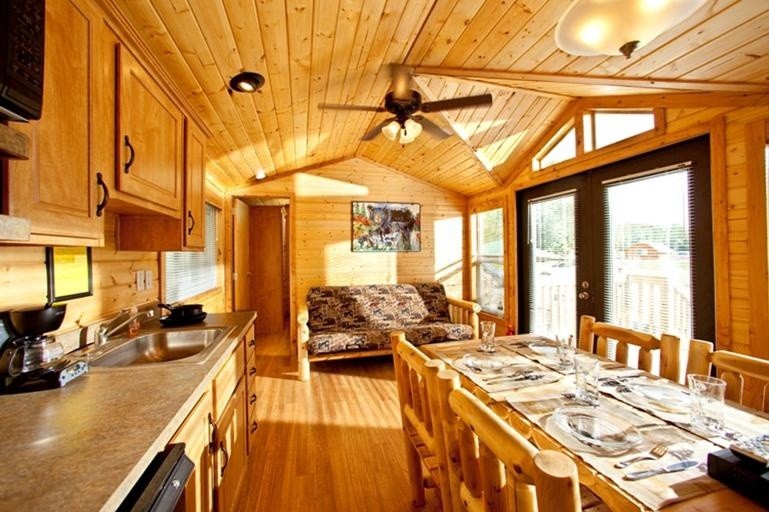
left=615, top=443, right=669, bottom=469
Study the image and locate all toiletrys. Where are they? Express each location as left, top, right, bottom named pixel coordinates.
left=128, top=304, right=138, bottom=327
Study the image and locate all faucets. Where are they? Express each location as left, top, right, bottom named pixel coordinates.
left=94, top=309, right=154, bottom=346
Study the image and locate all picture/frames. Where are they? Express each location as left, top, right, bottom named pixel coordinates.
left=45, top=246, right=93, bottom=302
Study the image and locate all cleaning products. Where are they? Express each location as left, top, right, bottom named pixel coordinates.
left=128, top=303, right=139, bottom=337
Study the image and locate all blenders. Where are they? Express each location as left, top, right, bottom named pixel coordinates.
left=8, top=305, right=67, bottom=378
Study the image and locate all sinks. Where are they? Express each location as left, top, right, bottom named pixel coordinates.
left=87, top=324, right=239, bottom=372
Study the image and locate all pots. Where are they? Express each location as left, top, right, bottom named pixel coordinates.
left=158, top=303, right=203, bottom=317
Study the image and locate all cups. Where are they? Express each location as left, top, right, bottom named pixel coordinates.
left=689, top=375, right=727, bottom=437
left=481, top=320, right=495, bottom=351
left=574, top=353, right=601, bottom=405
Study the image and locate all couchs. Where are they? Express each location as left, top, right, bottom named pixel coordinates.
left=297, top=283, right=481, bottom=383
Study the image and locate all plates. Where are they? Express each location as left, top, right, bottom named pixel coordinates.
left=628, top=382, right=691, bottom=409
left=555, top=407, right=643, bottom=456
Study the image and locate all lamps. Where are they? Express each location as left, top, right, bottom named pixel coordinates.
left=553, top=0, right=707, bottom=62
left=229, top=70, right=266, bottom=94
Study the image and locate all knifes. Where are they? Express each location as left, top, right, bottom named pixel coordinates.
left=626, top=461, right=699, bottom=480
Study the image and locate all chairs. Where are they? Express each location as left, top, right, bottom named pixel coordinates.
left=390, top=331, right=452, bottom=512
left=434, top=369, right=582, bottom=511
left=578, top=316, right=680, bottom=382
left=685, top=338, right=769, bottom=415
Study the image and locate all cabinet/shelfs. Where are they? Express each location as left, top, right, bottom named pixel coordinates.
left=106, top=29, right=184, bottom=222
left=244, top=323, right=260, bottom=455
left=170, top=389, right=214, bottom=512
left=116, top=116, right=206, bottom=253
left=213, top=341, right=248, bottom=512
left=4, top=0, right=110, bottom=248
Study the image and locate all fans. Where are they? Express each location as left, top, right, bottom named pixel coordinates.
left=318, top=88, right=492, bottom=141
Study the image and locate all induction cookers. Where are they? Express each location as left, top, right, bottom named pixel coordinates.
left=159, top=312, right=206, bottom=326
